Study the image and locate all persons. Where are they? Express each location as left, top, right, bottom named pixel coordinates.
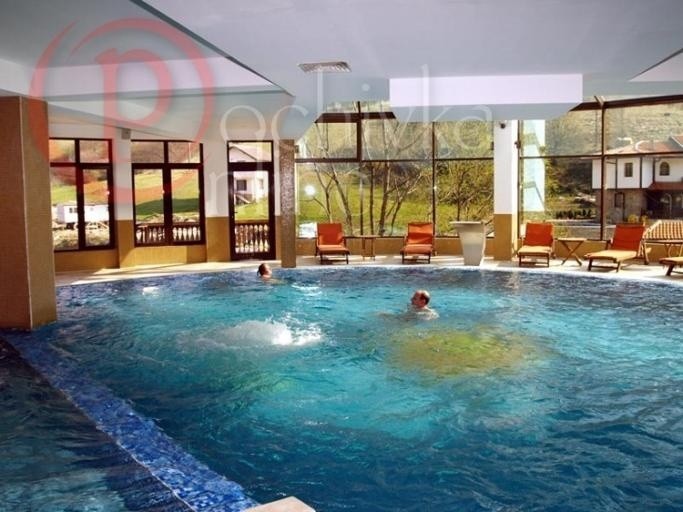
left=378, top=286, right=439, bottom=320
left=257, top=262, right=281, bottom=286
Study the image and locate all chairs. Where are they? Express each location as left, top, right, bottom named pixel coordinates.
left=518, top=219, right=555, bottom=270
left=400, top=220, right=435, bottom=265
left=583, top=225, right=647, bottom=272
left=313, top=222, right=350, bottom=264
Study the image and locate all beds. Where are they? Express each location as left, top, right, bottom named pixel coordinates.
left=343, top=234, right=380, bottom=265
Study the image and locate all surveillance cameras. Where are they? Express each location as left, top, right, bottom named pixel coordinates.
left=499, top=120, right=506, bottom=128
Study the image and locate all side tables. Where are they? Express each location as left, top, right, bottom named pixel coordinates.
left=661, top=240, right=683, bottom=271
left=559, top=238, right=584, bottom=266
